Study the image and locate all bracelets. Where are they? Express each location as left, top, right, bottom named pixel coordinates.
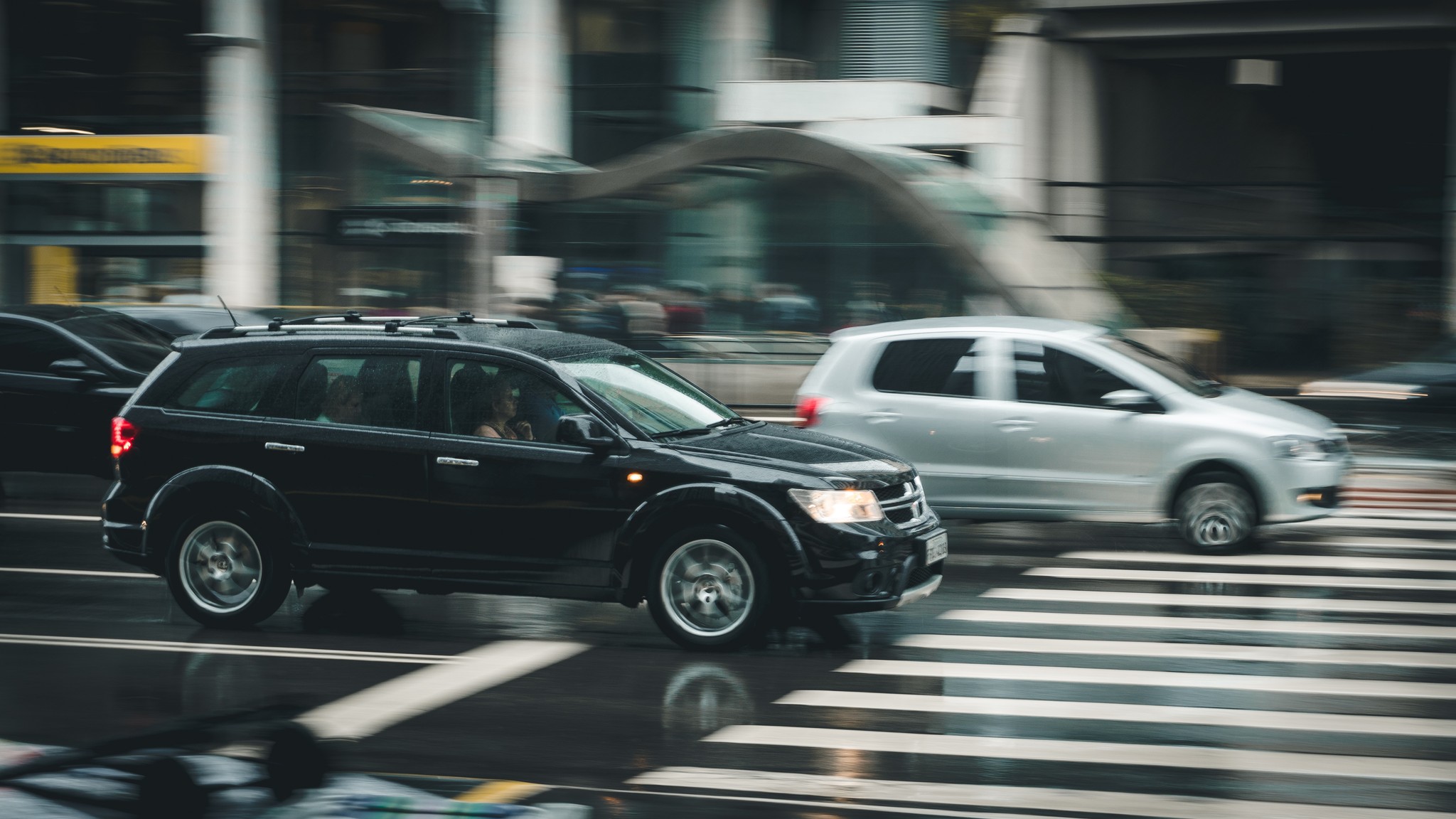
left=527, top=437, right=536, bottom=442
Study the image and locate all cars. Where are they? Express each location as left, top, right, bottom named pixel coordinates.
left=711, top=282, right=956, bottom=332
left=794, top=319, right=1347, bottom=553
left=0, top=298, right=276, bottom=469
left=489, top=290, right=704, bottom=339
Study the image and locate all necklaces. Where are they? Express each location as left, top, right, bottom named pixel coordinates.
left=488, top=419, right=518, bottom=433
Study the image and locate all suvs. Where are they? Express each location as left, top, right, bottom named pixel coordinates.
left=101, top=291, right=946, bottom=651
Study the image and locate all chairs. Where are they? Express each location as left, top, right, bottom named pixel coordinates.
left=254, top=355, right=537, bottom=437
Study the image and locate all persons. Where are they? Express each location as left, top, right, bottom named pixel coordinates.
left=524, top=374, right=572, bottom=445
left=471, top=374, right=538, bottom=442
left=315, top=374, right=373, bottom=426
